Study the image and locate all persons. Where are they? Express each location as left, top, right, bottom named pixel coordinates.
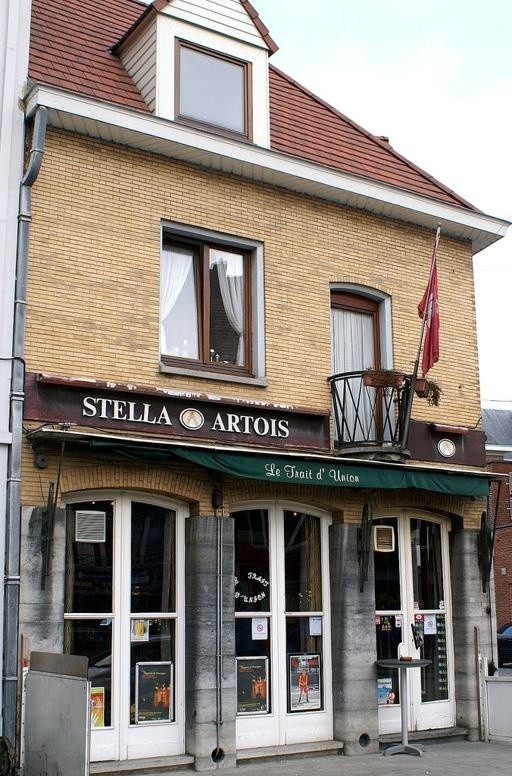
left=298, top=669, right=310, bottom=703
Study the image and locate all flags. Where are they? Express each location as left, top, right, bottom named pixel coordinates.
left=417, top=234, right=440, bottom=378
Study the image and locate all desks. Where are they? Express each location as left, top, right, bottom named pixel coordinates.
left=375, top=659, right=433, bottom=759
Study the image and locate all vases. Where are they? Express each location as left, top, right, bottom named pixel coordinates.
left=361, top=373, right=405, bottom=387
left=414, top=379, right=429, bottom=398
